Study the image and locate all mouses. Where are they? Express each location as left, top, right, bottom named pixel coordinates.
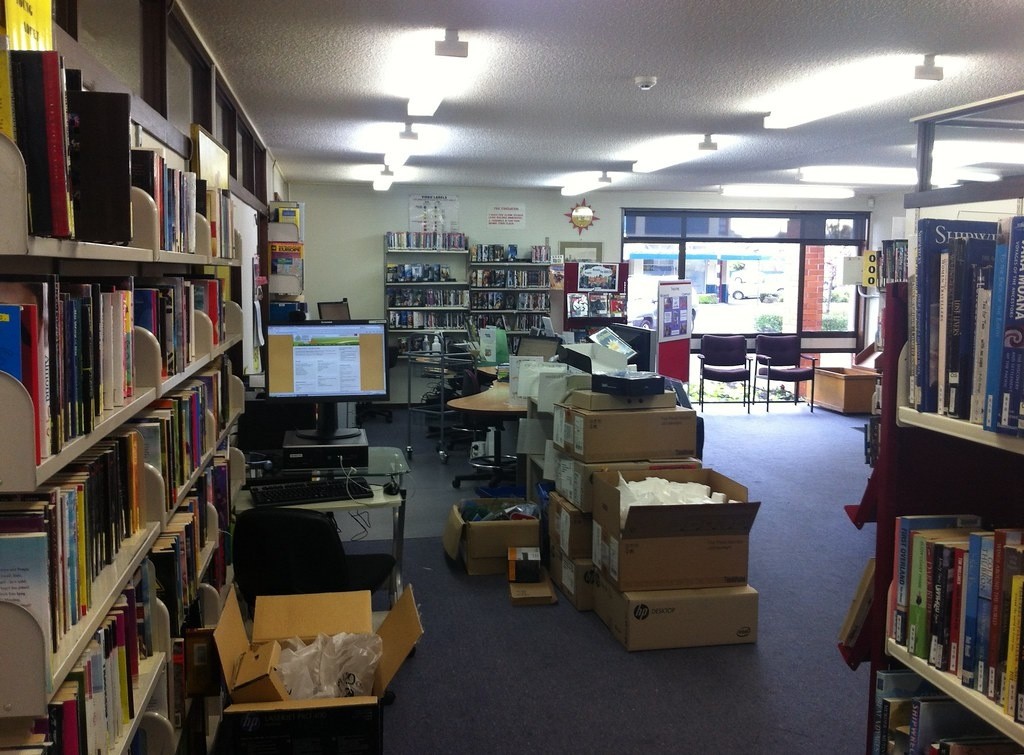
left=384, top=481, right=400, bottom=495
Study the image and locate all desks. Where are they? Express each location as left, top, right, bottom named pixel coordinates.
left=416, top=356, right=530, bottom=436
left=248, top=447, right=411, bottom=580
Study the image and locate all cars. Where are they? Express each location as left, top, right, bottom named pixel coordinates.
left=729, top=270, right=786, bottom=302
left=627, top=274, right=697, bottom=332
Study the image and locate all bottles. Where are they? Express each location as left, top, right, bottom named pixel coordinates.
left=432, top=336, right=441, bottom=356
left=423, top=335, right=429, bottom=352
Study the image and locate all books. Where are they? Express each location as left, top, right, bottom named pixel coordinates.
left=871, top=671, right=1024, bottom=755
left=386, top=288, right=551, bottom=310
left=469, top=244, right=504, bottom=262
left=0, top=275, right=231, bottom=755
left=876, top=240, right=908, bottom=287
left=271, top=242, right=303, bottom=295
left=470, top=270, right=550, bottom=287
left=837, top=556, right=875, bottom=648
left=396, top=337, right=423, bottom=351
left=895, top=513, right=1024, bottom=725
left=387, top=263, right=456, bottom=282
left=508, top=244, right=517, bottom=262
left=506, top=336, right=522, bottom=356
left=567, top=292, right=626, bottom=317
left=390, top=310, right=551, bottom=330
left=549, top=255, right=564, bottom=288
left=531, top=246, right=550, bottom=263
left=908, top=216, right=1024, bottom=436
left=387, top=231, right=465, bottom=251
left=0, top=50, right=236, bottom=260
left=497, top=363, right=509, bottom=380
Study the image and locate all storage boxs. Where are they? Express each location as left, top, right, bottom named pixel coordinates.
left=477, top=342, right=761, bottom=653
left=805, top=342, right=883, bottom=417
left=211, top=582, right=425, bottom=755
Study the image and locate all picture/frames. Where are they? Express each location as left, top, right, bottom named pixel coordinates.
left=189, top=122, right=231, bottom=190
left=578, top=261, right=619, bottom=293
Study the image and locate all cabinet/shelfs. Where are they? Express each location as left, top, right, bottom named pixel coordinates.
left=471, top=262, right=553, bottom=359
left=865, top=282, right=1024, bottom=755
left=383, top=234, right=470, bottom=367
left=0, top=0, right=273, bottom=754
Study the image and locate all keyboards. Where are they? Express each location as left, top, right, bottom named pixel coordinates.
left=249, top=476, right=374, bottom=507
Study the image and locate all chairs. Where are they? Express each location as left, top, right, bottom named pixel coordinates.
left=451, top=368, right=516, bottom=487
left=698, top=331, right=754, bottom=416
left=230, top=509, right=397, bottom=620
left=758, top=331, right=819, bottom=414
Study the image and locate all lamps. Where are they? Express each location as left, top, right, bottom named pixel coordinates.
left=406, top=26, right=469, bottom=121
left=763, top=52, right=958, bottom=130
left=558, top=169, right=613, bottom=198
left=383, top=127, right=423, bottom=169
left=631, top=131, right=720, bottom=177
left=365, top=171, right=398, bottom=192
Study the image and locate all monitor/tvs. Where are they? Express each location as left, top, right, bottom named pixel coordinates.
left=515, top=335, right=563, bottom=362
left=529, top=326, right=540, bottom=336
left=262, top=319, right=391, bottom=440
left=269, top=301, right=299, bottom=322
left=610, top=323, right=656, bottom=371
left=317, top=302, right=351, bottom=320
